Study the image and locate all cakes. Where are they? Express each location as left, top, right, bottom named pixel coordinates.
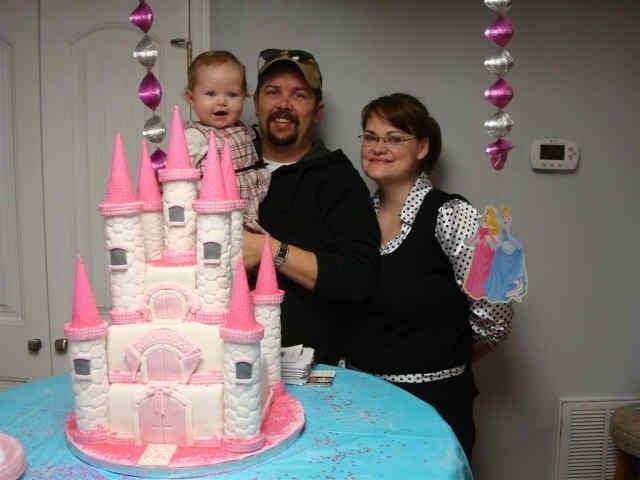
left=63, top=106, right=285, bottom=453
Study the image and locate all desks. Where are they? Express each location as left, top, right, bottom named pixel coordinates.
left=0, top=364, right=469, bottom=480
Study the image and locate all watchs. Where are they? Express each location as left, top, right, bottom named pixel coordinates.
left=273, top=240, right=288, bottom=269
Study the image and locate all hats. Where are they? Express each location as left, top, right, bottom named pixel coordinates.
left=258, top=48, right=322, bottom=91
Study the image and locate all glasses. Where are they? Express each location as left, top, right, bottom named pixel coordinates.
left=357, top=134, right=416, bottom=145
left=260, top=49, right=313, bottom=62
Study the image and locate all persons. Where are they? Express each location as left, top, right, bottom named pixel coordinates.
left=326, top=94, right=515, bottom=467
left=241, top=48, right=380, bottom=367
left=183, top=50, right=272, bottom=237
